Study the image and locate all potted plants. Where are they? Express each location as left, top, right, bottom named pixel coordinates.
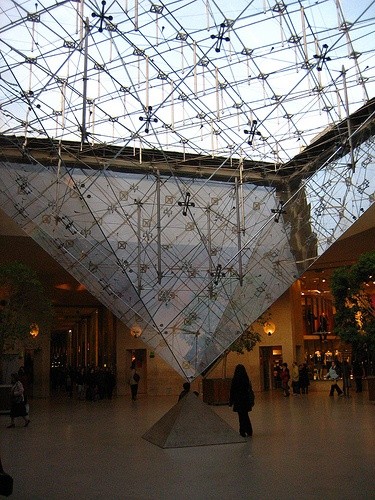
left=180, top=301, right=271, bottom=406
left=330, top=250, right=375, bottom=401
left=0, top=262, right=61, bottom=415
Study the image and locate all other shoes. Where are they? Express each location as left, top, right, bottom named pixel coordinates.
left=285, top=394, right=289, bottom=397
left=24, top=420, right=30, bottom=427
left=240, top=433, right=247, bottom=437
left=7, top=425, right=15, bottom=428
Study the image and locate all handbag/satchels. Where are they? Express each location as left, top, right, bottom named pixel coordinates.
left=12, top=384, right=23, bottom=403
left=0, top=461, right=13, bottom=496
left=133, top=371, right=140, bottom=382
left=248, top=383, right=254, bottom=404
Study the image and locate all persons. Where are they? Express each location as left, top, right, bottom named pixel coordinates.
left=320, top=315, right=329, bottom=332
left=7, top=373, right=31, bottom=428
left=229, top=364, right=252, bottom=437
left=308, top=310, right=317, bottom=333
left=325, top=361, right=343, bottom=396
left=20, top=366, right=28, bottom=405
left=64, top=366, right=74, bottom=399
left=352, top=361, right=363, bottom=392
left=341, top=361, right=351, bottom=396
left=127, top=365, right=140, bottom=400
left=274, top=363, right=310, bottom=397
left=77, top=367, right=117, bottom=402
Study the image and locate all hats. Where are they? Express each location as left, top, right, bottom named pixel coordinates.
left=282, top=363, right=287, bottom=366
